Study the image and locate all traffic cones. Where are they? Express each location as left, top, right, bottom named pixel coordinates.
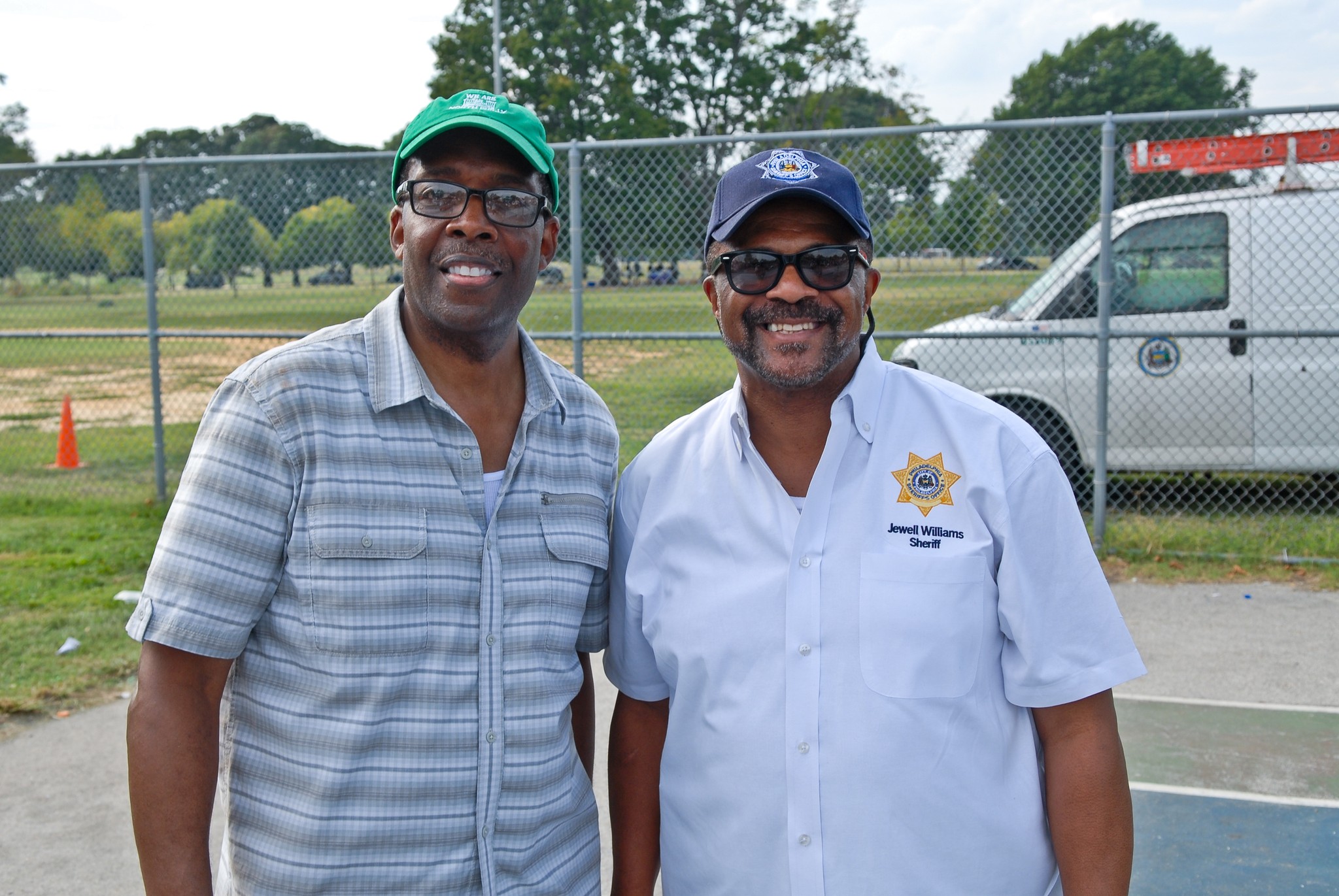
left=42, top=396, right=88, bottom=469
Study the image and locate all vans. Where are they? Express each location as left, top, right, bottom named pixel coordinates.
left=889, top=178, right=1339, bottom=510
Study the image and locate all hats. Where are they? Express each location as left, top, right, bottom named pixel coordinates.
left=703, top=148, right=874, bottom=261
left=391, top=88, right=558, bottom=214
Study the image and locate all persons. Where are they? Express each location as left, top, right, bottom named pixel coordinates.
left=624, top=259, right=678, bottom=283
left=601, top=146, right=1149, bottom=896
left=126, top=89, right=620, bottom=896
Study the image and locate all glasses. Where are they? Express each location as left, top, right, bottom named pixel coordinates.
left=396, top=178, right=554, bottom=229
left=709, top=245, right=870, bottom=295
